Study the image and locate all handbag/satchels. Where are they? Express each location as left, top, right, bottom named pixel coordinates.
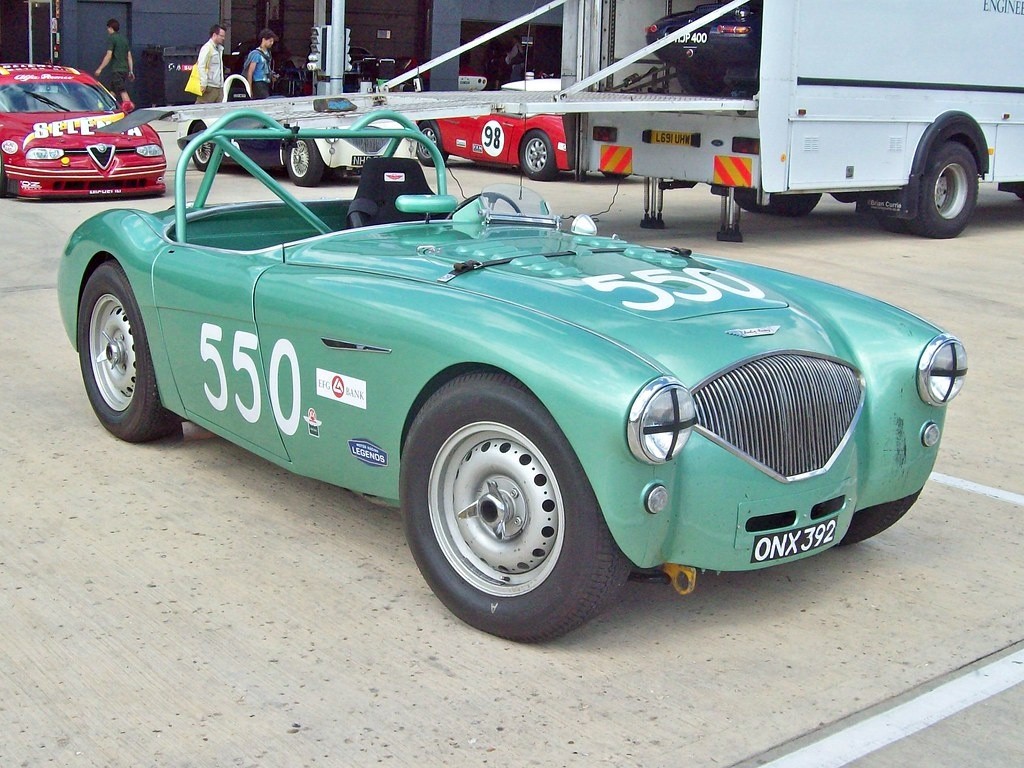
left=185, top=47, right=212, bottom=96
left=511, top=45, right=524, bottom=65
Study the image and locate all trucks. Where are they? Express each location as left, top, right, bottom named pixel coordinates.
left=144, top=0, right=1023, bottom=240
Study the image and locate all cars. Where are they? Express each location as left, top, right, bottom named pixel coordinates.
left=177, top=94, right=419, bottom=187
left=414, top=78, right=632, bottom=181
left=0, top=58, right=167, bottom=204
left=643, top=0, right=762, bottom=96
left=55, top=107, right=969, bottom=646
left=395, top=54, right=486, bottom=93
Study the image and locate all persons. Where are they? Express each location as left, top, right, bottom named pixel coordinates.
left=94, top=19, right=137, bottom=112
left=483, top=38, right=506, bottom=90
left=243, top=28, right=280, bottom=100
left=506, top=35, right=525, bottom=82
left=193, top=22, right=229, bottom=103
